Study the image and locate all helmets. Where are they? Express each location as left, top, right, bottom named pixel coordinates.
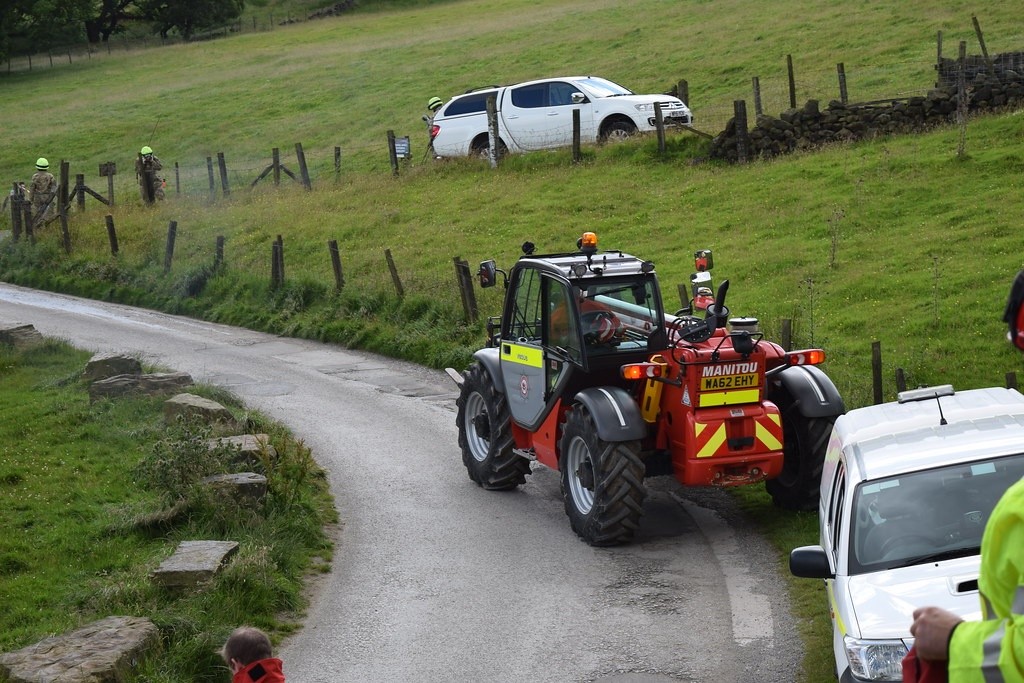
left=141, top=145, right=153, bottom=155
left=428, top=97, right=443, bottom=110
left=36, top=157, right=50, bottom=170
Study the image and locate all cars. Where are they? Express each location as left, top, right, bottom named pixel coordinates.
left=789, top=385, right=1024, bottom=683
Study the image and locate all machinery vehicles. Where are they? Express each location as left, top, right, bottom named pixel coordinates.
left=444, top=232, right=845, bottom=547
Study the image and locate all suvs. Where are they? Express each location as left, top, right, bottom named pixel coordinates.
left=432, top=76, right=694, bottom=162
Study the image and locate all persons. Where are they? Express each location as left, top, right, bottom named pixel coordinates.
left=134, top=146, right=167, bottom=204
left=909, top=471, right=1024, bottom=683
left=28, top=157, right=57, bottom=228
left=548, top=292, right=621, bottom=345
left=220, top=626, right=286, bottom=683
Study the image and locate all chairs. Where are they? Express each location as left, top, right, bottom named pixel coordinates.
left=863, top=482, right=933, bottom=562
